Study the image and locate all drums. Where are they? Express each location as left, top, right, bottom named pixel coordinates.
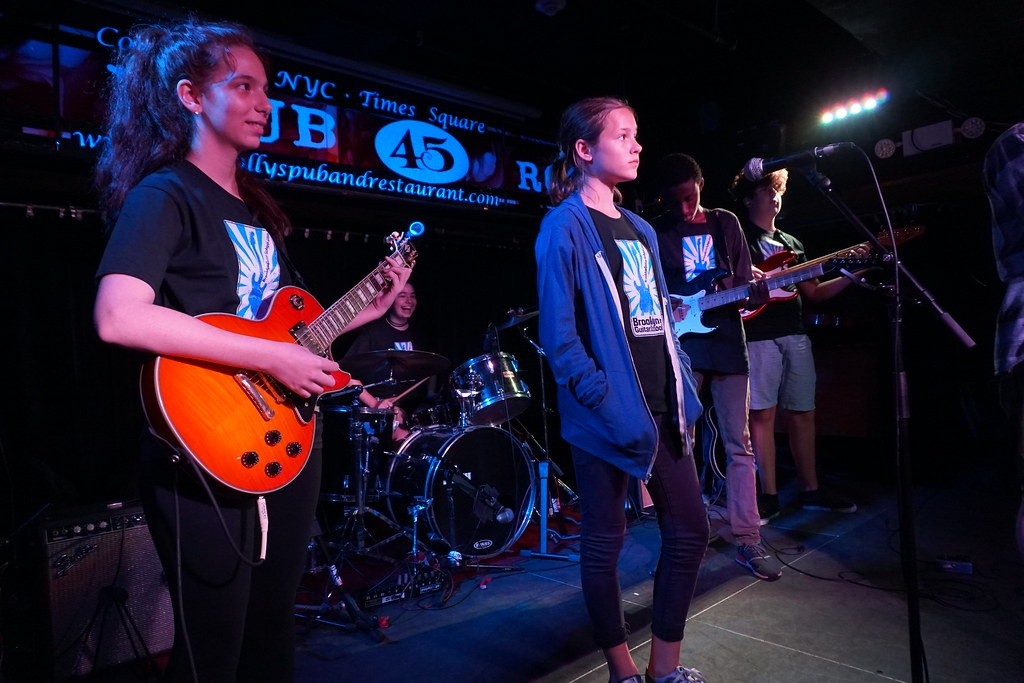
left=407, top=399, right=460, bottom=425
left=321, top=406, right=399, bottom=499
left=448, top=352, right=532, bottom=426
left=384, top=424, right=538, bottom=561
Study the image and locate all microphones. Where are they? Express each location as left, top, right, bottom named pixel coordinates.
left=497, top=508, right=514, bottom=524
left=99, top=585, right=129, bottom=604
left=744, top=142, right=857, bottom=182
left=484, top=322, right=494, bottom=352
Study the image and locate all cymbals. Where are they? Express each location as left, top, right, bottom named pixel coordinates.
left=479, top=310, right=539, bottom=337
left=336, top=349, right=452, bottom=382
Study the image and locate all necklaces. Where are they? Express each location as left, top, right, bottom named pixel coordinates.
left=387, top=317, right=407, bottom=327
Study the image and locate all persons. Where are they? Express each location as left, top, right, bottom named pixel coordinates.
left=979, top=121, right=1024, bottom=549
left=338, top=281, right=430, bottom=441
left=92, top=18, right=411, bottom=683
left=535, top=98, right=710, bottom=683
left=642, top=154, right=783, bottom=581
left=729, top=162, right=883, bottom=526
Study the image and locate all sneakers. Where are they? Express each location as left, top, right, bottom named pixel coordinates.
left=709, top=527, right=720, bottom=545
left=759, top=493, right=781, bottom=526
left=735, top=543, right=782, bottom=580
left=800, top=489, right=857, bottom=513
left=645, top=664, right=708, bottom=683
left=608, top=674, right=644, bottom=683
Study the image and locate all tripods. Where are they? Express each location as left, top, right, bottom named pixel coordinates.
left=305, top=364, right=524, bottom=601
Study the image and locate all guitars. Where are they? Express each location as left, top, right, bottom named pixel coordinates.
left=706, top=404, right=727, bottom=481
left=739, top=249, right=882, bottom=321
left=669, top=224, right=927, bottom=339
left=138, top=229, right=419, bottom=502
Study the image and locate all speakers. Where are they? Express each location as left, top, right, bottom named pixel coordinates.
left=1, top=502, right=175, bottom=683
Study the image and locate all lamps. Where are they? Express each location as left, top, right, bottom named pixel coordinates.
left=874, top=118, right=985, bottom=158
